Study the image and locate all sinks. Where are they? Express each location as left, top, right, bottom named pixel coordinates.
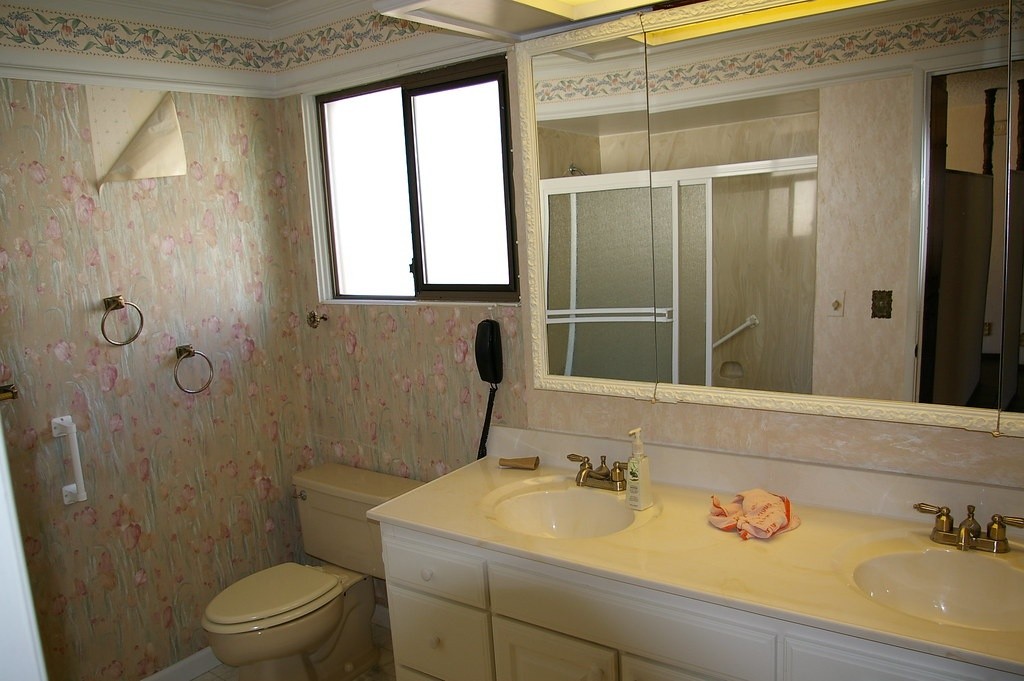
left=848, top=548, right=1024, bottom=632
left=493, top=483, right=640, bottom=543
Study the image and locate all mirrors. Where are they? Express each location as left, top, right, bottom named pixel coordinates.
left=514, top=0, right=1024, bottom=438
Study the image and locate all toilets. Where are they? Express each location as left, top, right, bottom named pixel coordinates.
left=200, top=464, right=429, bottom=680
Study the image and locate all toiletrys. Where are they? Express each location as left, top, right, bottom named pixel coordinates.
left=625, top=429, right=653, bottom=510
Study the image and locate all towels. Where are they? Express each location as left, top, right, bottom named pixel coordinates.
left=708, top=489, right=791, bottom=541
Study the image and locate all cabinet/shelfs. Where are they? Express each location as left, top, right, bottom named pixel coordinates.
left=379, top=521, right=492, bottom=681
left=491, top=615, right=707, bottom=681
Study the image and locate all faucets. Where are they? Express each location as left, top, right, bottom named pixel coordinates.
left=957, top=504, right=982, bottom=550
left=576, top=455, right=610, bottom=487
